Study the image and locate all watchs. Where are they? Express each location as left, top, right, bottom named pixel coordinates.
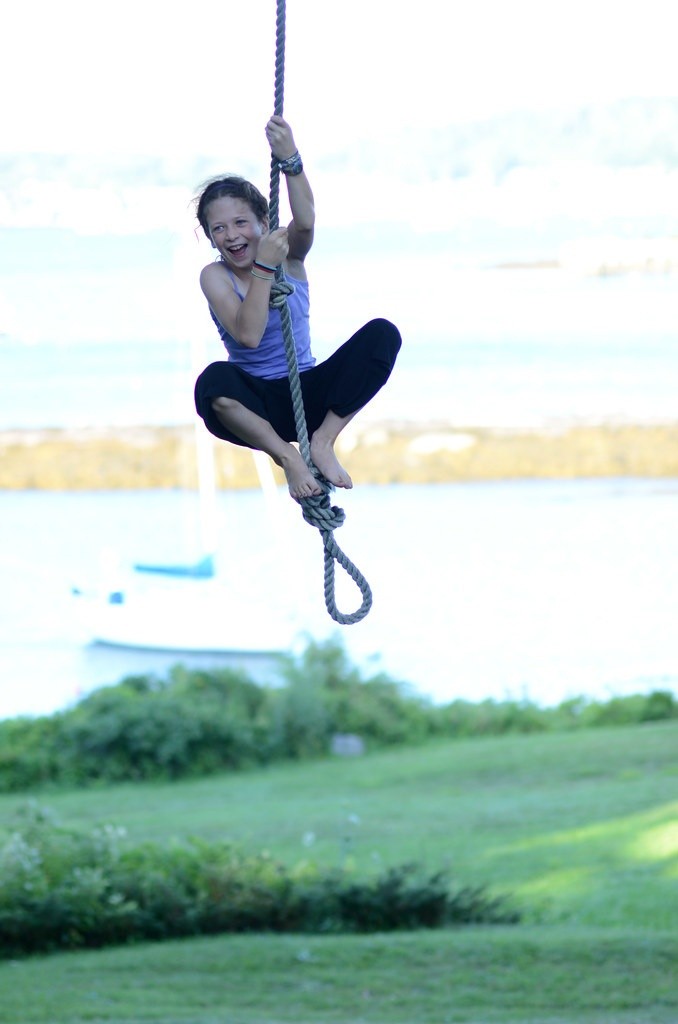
left=283, top=162, right=304, bottom=175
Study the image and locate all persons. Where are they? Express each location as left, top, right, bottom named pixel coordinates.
left=193, top=113, right=402, bottom=498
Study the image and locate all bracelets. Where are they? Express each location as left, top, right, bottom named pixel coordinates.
left=278, top=151, right=301, bottom=167
left=251, top=259, right=278, bottom=280
